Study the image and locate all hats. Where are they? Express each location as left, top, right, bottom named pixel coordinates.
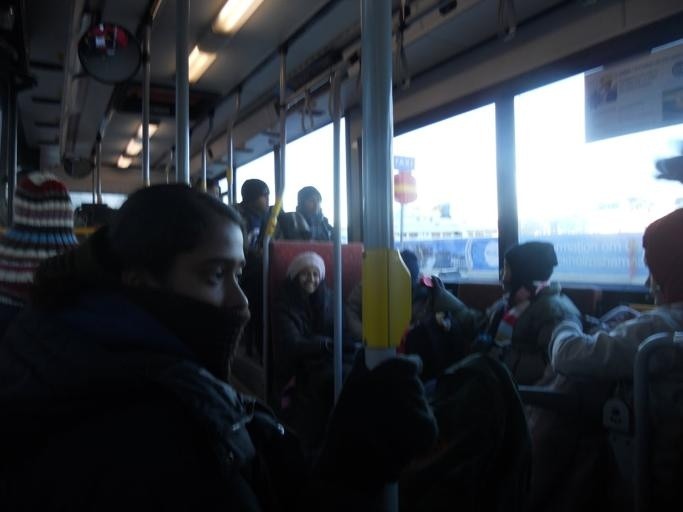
left=286, top=251, right=328, bottom=282
left=0, top=172, right=83, bottom=307
left=503, top=239, right=559, bottom=282
left=642, top=207, right=682, bottom=298
left=240, top=178, right=271, bottom=201
left=296, top=185, right=322, bottom=204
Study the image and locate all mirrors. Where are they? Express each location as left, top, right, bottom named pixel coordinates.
left=78, top=21, right=143, bottom=86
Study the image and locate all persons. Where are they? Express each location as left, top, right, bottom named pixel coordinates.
left=546, top=208, right=683, bottom=511
left=279, top=241, right=583, bottom=511
left=207, top=179, right=335, bottom=342
left=2, top=183, right=299, bottom=503
left=2, top=172, right=106, bottom=331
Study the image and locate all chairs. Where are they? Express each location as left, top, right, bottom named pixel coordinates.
left=268, top=236, right=598, bottom=332
